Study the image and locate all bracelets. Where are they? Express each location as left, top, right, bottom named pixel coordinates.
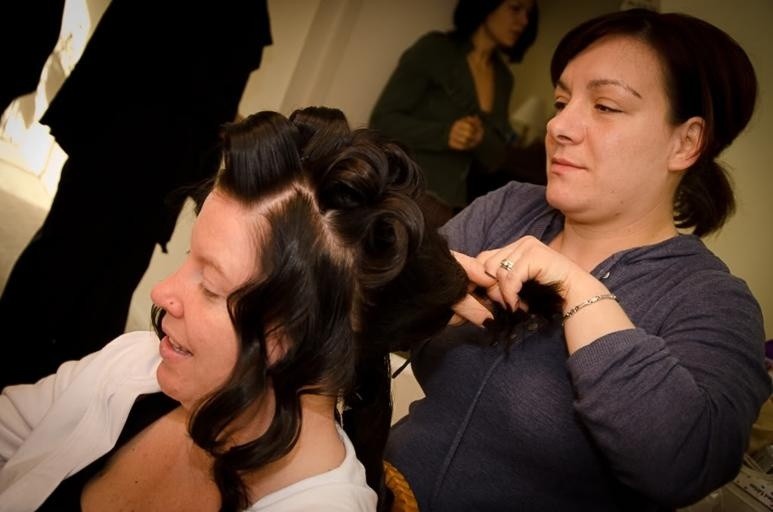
left=560, top=291, right=618, bottom=329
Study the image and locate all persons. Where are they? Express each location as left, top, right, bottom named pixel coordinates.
left=367, top=0, right=540, bottom=228
left=1, top=0, right=273, bottom=391
left=1, top=107, right=470, bottom=512
left=379, top=11, right=765, bottom=509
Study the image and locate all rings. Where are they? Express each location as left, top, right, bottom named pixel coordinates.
left=500, top=258, right=514, bottom=271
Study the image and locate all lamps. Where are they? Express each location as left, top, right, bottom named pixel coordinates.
left=511, top=98, right=547, bottom=144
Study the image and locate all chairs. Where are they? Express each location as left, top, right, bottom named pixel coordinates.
left=379, top=455, right=419, bottom=511
